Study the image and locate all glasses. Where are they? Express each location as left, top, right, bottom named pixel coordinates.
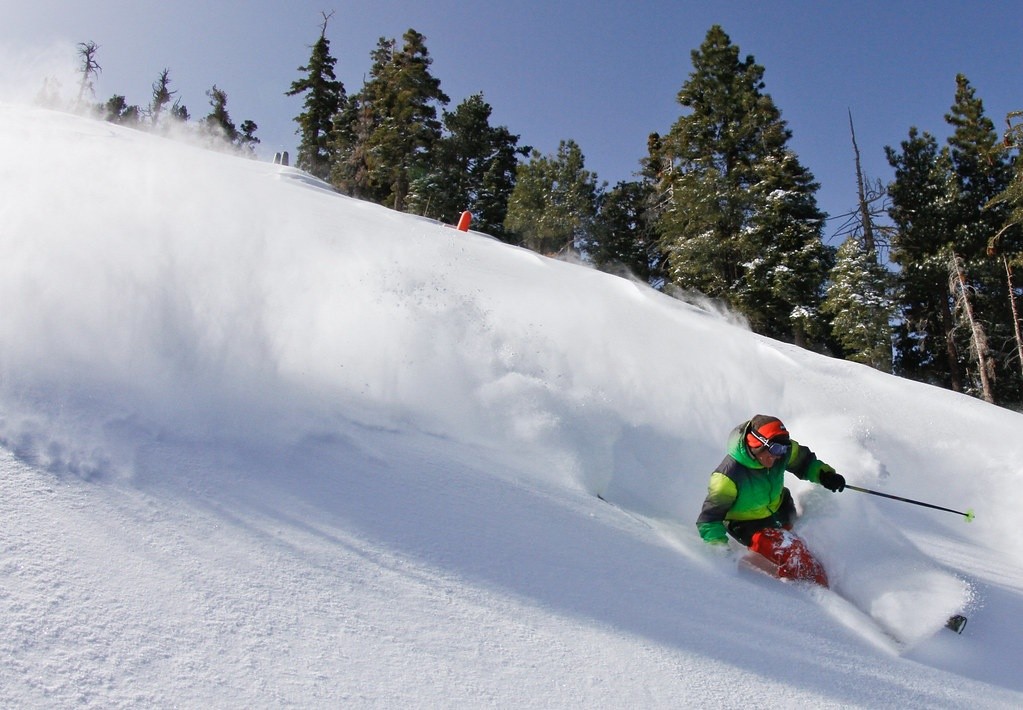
left=769, top=443, right=790, bottom=458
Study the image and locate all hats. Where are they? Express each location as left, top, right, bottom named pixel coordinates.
left=747, top=415, right=791, bottom=455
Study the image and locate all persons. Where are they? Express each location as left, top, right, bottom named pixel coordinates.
left=695, top=415, right=846, bottom=590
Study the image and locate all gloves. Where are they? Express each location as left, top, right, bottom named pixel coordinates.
left=820, top=470, right=845, bottom=494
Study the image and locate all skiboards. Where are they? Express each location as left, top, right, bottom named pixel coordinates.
left=594, top=489, right=968, bottom=645
